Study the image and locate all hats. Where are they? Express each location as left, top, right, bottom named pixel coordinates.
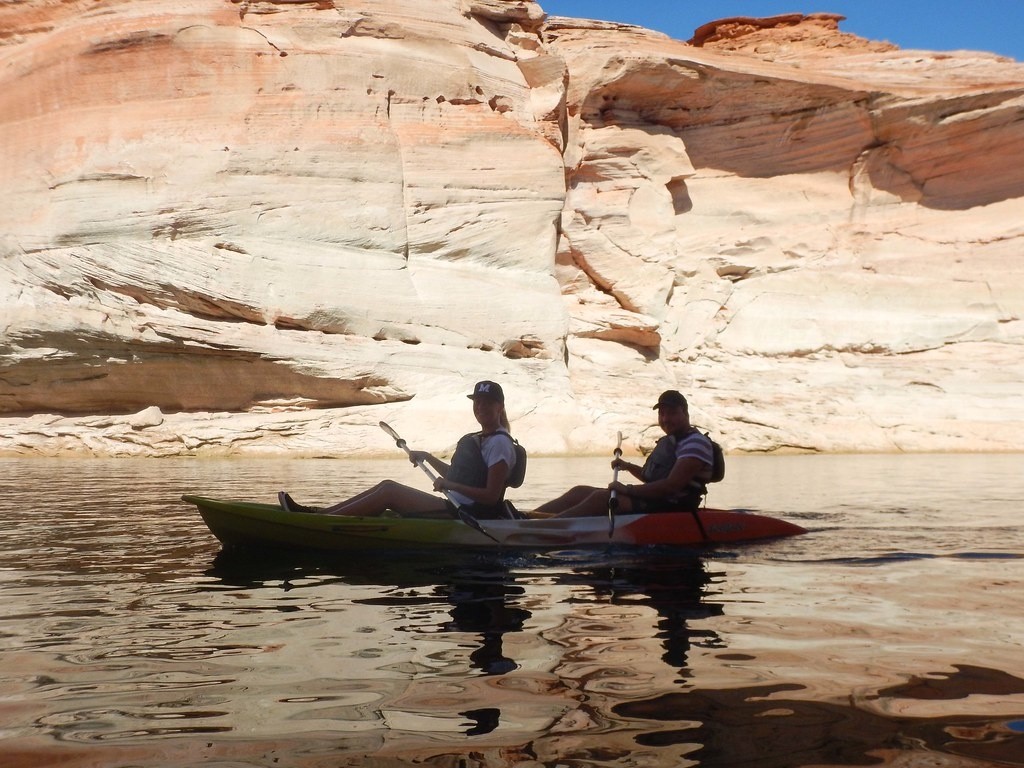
left=653, top=390, right=686, bottom=410
left=466, top=381, right=503, bottom=403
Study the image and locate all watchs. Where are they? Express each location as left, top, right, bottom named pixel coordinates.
left=627, top=484, right=634, bottom=496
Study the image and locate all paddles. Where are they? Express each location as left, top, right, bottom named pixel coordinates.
left=608, top=430, right=623, bottom=539
left=379, top=420, right=501, bottom=544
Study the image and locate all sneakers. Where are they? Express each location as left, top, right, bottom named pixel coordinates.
left=278, top=491, right=285, bottom=510
left=280, top=493, right=317, bottom=513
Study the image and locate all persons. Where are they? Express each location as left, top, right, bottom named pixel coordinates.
left=503, top=389, right=714, bottom=520
left=278, top=381, right=518, bottom=520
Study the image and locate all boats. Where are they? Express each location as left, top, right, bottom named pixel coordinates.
left=181, top=492, right=811, bottom=558
